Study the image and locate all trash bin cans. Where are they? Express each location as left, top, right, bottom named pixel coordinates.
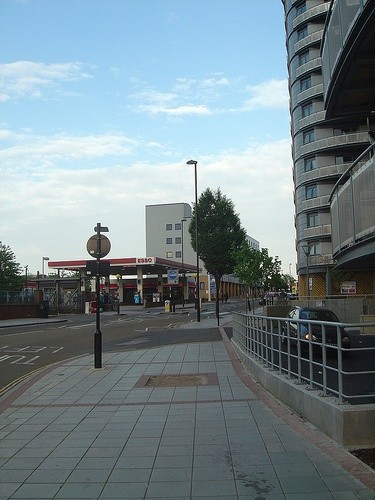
left=39, top=300, right=49, bottom=318
left=164, top=300, right=170, bottom=312
left=85, top=302, right=92, bottom=315
left=92, top=301, right=97, bottom=314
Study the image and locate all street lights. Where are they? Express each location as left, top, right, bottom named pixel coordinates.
left=289, top=262, right=293, bottom=278
left=185, top=159, right=201, bottom=322
left=181, top=219, right=187, bottom=267
left=42, top=257, right=50, bottom=279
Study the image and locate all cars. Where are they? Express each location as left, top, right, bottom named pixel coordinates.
left=259, top=291, right=293, bottom=306
left=280, top=305, right=351, bottom=357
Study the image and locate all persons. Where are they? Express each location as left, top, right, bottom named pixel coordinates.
left=224, top=291, right=228, bottom=303
left=165, top=297, right=176, bottom=312
left=317, top=311, right=331, bottom=321
left=289, top=294, right=299, bottom=300
